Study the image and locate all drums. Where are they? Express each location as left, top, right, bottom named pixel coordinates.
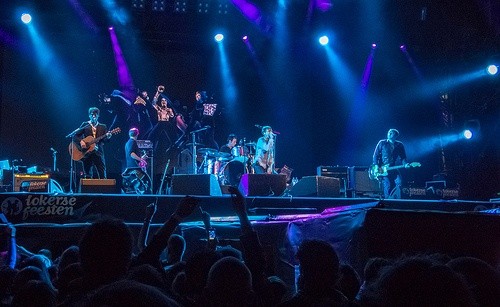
left=232, top=156, right=249, bottom=163
left=218, top=161, right=247, bottom=186
left=207, top=158, right=230, bottom=174
left=230, top=146, right=249, bottom=156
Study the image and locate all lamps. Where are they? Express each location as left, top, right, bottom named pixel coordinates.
left=240, top=32, right=247, bottom=40
left=463, top=119, right=483, bottom=141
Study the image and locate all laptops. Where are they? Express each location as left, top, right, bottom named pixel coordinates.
left=0, top=160, right=11, bottom=169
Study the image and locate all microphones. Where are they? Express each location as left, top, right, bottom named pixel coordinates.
left=255, top=124, right=260, bottom=127
left=205, top=126, right=211, bottom=128
left=272, top=134, right=277, bottom=136
left=51, top=148, right=57, bottom=153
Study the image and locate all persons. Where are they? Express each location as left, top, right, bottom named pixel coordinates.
left=0, top=186, right=500, bottom=307
left=65, top=86, right=275, bottom=193
left=373, top=129, right=410, bottom=199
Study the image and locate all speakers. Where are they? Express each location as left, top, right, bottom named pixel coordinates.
left=172, top=174, right=222, bottom=195
left=238, top=174, right=286, bottom=197
left=80, top=178, right=122, bottom=194
left=389, top=186, right=459, bottom=200
left=288, top=166, right=382, bottom=198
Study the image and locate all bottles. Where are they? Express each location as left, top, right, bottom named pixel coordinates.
left=204, top=163, right=208, bottom=174
left=292, top=177, right=298, bottom=186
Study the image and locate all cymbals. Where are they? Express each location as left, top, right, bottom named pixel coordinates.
left=208, top=152, right=230, bottom=158
left=197, top=148, right=217, bottom=153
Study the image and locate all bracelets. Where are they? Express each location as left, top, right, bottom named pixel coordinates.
left=9, top=234, right=15, bottom=238
left=171, top=211, right=183, bottom=223
left=144, top=219, right=151, bottom=222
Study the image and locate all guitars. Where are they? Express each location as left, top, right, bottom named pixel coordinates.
left=367, top=161, right=421, bottom=181
left=68, top=127, right=121, bottom=163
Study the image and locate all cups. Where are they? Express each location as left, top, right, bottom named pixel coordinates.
left=209, top=228, right=216, bottom=239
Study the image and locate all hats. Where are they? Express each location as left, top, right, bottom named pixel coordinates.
left=129, top=128, right=139, bottom=137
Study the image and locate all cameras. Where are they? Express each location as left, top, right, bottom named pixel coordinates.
left=208, top=228, right=216, bottom=240
